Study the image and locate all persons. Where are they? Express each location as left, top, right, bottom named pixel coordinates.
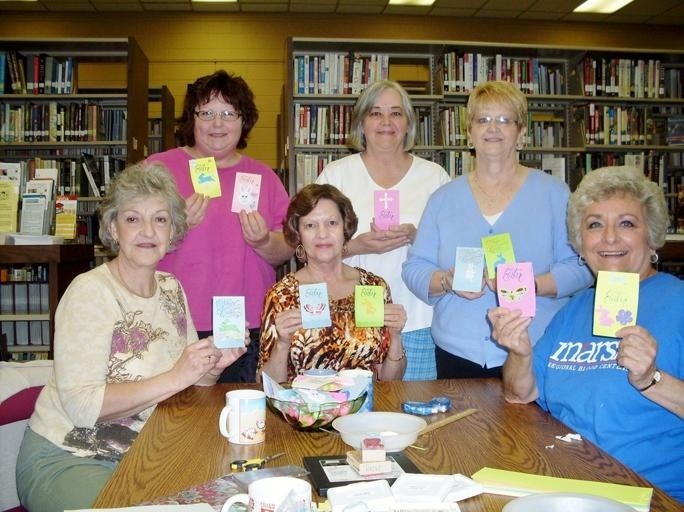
left=137, top=70, right=297, bottom=383
left=400, top=77, right=597, bottom=381
left=253, top=183, right=408, bottom=382
left=315, top=77, right=453, bottom=380
left=13, top=159, right=251, bottom=511
left=486, top=166, right=684, bottom=504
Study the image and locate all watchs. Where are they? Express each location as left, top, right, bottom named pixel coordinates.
left=638, top=368, right=663, bottom=392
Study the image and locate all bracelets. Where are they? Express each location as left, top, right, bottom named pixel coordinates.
left=387, top=347, right=407, bottom=361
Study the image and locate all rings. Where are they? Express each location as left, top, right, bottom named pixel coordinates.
left=208, top=356, right=211, bottom=363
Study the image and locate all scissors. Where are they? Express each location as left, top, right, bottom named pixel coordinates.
left=230, top=452, right=284, bottom=472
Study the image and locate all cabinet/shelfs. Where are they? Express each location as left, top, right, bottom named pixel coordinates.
left=1, top=246, right=93, bottom=363
left=79, top=85, right=176, bottom=158
left=0, top=38, right=149, bottom=265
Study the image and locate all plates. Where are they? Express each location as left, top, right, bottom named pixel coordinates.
left=500, top=491, right=639, bottom=512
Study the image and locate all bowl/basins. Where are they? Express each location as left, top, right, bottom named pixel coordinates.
left=332, top=412, right=428, bottom=452
left=266, top=382, right=368, bottom=432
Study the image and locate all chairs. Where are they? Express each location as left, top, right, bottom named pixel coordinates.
left=0, top=386, right=44, bottom=512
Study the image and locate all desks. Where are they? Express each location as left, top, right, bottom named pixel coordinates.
left=94, top=378, right=684, bottom=512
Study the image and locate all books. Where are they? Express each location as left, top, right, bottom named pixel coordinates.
left=581, top=56, right=684, bottom=233
left=443, top=52, right=566, bottom=180
left=469, top=466, right=655, bottom=512
left=293, top=49, right=432, bottom=192
left=1, top=50, right=162, bottom=360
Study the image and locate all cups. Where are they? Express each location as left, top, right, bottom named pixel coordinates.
left=303, top=369, right=336, bottom=378
left=218, top=389, right=266, bottom=446
left=221, top=476, right=312, bottom=512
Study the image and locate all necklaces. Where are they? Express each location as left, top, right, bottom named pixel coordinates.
left=116, top=257, right=156, bottom=298
left=472, top=162, right=520, bottom=207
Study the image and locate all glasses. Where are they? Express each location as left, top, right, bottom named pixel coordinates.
left=471, top=118, right=516, bottom=130
left=194, top=107, right=241, bottom=122
